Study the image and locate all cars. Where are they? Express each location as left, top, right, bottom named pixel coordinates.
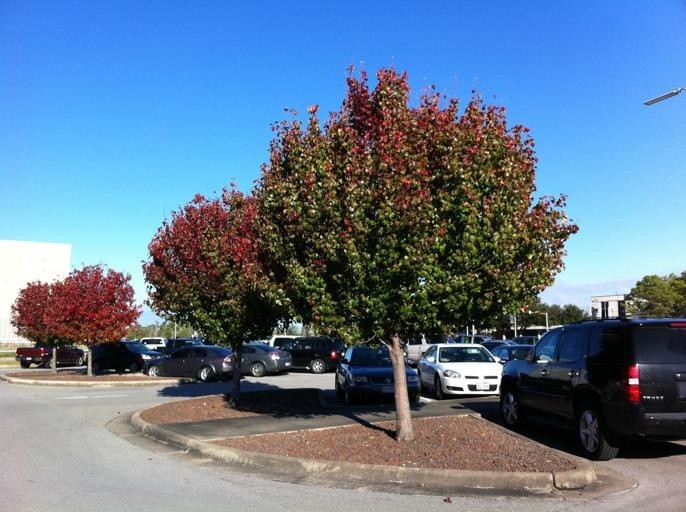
left=416, top=342, right=504, bottom=401
left=333, top=340, right=422, bottom=404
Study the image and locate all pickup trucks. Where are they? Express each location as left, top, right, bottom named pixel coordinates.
left=14, top=340, right=84, bottom=368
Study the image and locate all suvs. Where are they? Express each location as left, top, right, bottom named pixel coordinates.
left=498, top=315, right=686, bottom=462
